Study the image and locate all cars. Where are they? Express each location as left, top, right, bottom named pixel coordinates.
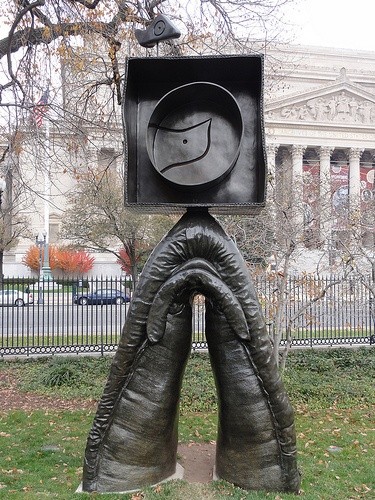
left=1, top=290, right=35, bottom=307
left=74, top=288, right=130, bottom=306
